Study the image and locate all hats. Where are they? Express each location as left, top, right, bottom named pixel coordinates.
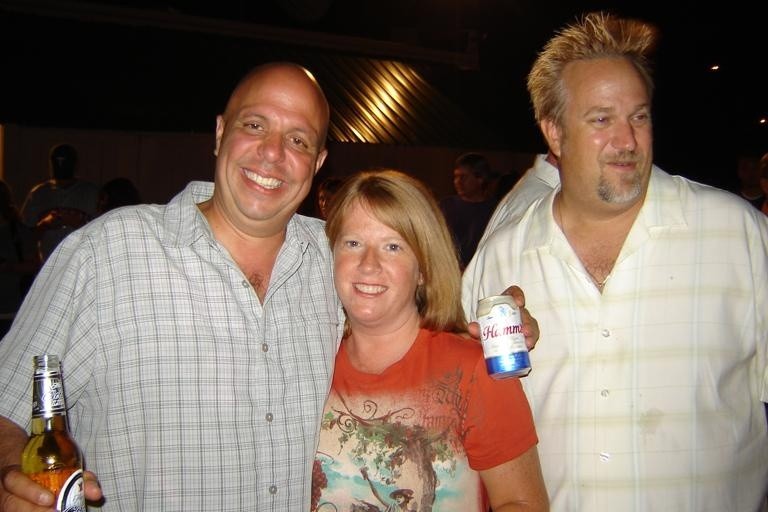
left=48, top=142, right=79, bottom=181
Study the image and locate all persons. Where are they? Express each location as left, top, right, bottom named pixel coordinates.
left=0, top=143, right=143, bottom=341
left=735, top=152, right=768, bottom=216
left=475, top=148, right=561, bottom=250
left=311, top=170, right=552, bottom=512
left=442, top=151, right=498, bottom=270
left=318, top=175, right=343, bottom=220
left=0, top=60, right=540, bottom=512
left=459, top=13, right=768, bottom=511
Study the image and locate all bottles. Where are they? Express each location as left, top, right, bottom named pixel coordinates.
left=17, top=353, right=88, bottom=512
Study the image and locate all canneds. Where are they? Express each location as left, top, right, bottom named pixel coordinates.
left=475, top=294, right=533, bottom=381
